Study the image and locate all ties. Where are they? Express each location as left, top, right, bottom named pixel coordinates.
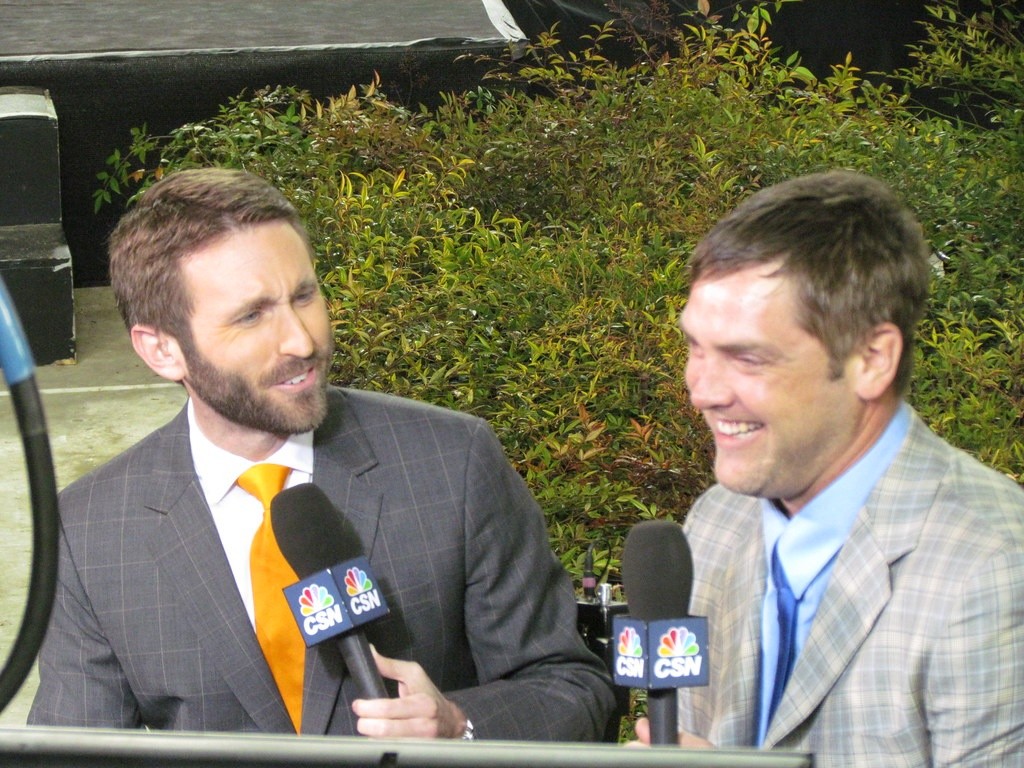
left=760, top=538, right=803, bottom=740
left=236, top=462, right=307, bottom=737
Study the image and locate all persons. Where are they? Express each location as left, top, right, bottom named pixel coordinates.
left=24, top=165, right=621, bottom=744
left=621, top=170, right=1024, bottom=767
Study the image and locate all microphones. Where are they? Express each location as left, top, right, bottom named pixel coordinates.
left=612, top=520, right=710, bottom=748
left=270, top=482, right=391, bottom=702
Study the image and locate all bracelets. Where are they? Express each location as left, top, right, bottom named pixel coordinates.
left=462, top=718, right=475, bottom=742
